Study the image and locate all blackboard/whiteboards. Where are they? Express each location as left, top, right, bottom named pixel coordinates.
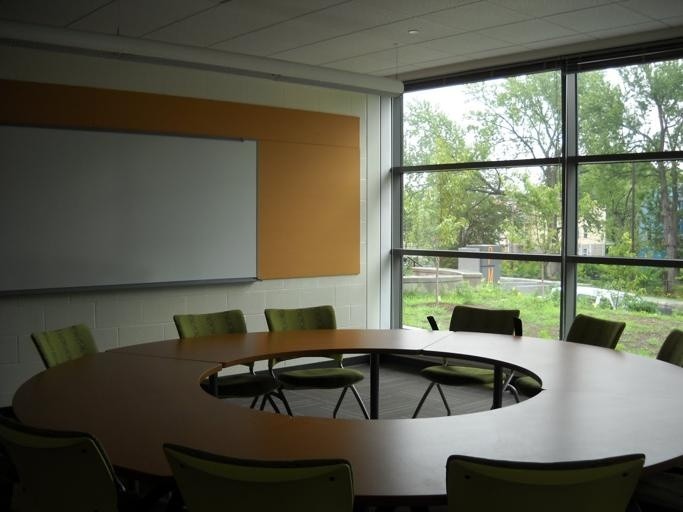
left=0, top=124, right=258, bottom=298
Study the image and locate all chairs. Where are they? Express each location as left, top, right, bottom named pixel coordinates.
left=32, top=325, right=99, bottom=370
left=162, top=442, right=355, bottom=510
left=260, top=307, right=370, bottom=421
left=446, top=454, right=644, bottom=509
left=658, top=328, right=683, bottom=366
left=412, top=306, right=522, bottom=421
left=174, top=310, right=281, bottom=411
left=3, top=404, right=133, bottom=509
left=567, top=315, right=625, bottom=351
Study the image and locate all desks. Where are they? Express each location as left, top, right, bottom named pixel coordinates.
left=3, top=328, right=683, bottom=499
